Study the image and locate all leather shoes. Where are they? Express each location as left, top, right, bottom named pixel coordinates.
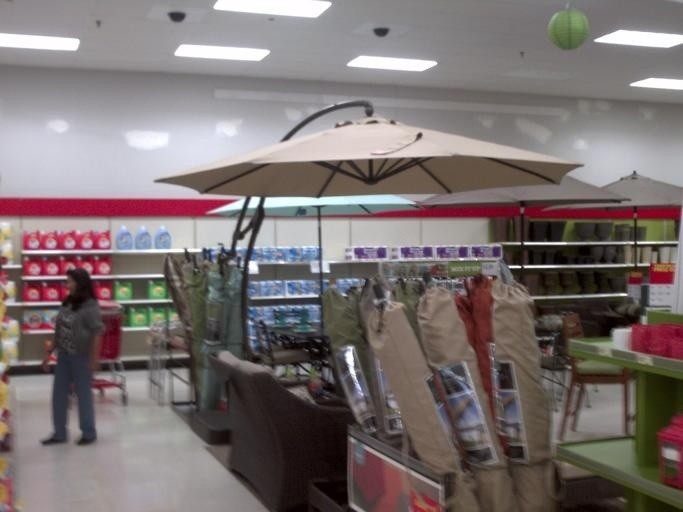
left=42, top=438, right=63, bottom=444
left=76, top=436, right=97, bottom=445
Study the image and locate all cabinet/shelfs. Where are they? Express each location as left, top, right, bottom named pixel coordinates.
left=0, top=241, right=683, bottom=512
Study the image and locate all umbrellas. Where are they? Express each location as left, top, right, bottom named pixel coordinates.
left=164, top=242, right=242, bottom=413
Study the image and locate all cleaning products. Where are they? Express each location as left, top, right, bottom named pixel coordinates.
left=23, top=227, right=181, bottom=331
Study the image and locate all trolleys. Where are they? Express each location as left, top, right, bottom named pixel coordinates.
left=49, top=299, right=130, bottom=405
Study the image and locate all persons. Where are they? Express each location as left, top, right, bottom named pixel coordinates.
left=39, top=268, right=106, bottom=446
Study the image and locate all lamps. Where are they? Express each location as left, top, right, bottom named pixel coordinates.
left=547, top=0, right=588, bottom=51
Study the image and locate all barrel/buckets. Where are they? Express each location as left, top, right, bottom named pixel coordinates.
left=655, top=414, right=683, bottom=491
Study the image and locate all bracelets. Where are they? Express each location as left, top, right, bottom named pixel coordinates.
left=46, top=348, right=51, bottom=355
